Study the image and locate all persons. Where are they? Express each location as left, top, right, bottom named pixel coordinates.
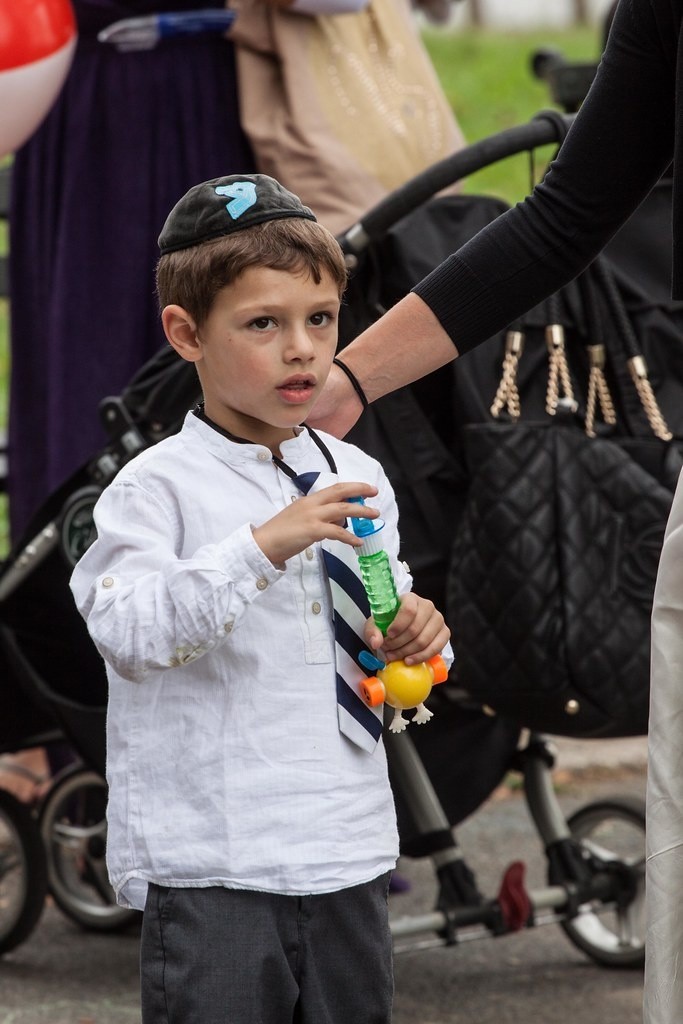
left=69, top=174, right=454, bottom=1024
left=300, top=0, right=683, bottom=1024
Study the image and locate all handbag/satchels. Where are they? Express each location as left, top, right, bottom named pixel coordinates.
left=227, top=0, right=470, bottom=239
left=442, top=412, right=683, bottom=737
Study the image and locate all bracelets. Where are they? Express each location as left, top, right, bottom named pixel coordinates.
left=332, top=357, right=368, bottom=409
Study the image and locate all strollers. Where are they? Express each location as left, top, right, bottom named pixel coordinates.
left=0, top=95, right=649, bottom=971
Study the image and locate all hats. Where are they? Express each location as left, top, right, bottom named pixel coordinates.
left=158, top=173, right=316, bottom=255
